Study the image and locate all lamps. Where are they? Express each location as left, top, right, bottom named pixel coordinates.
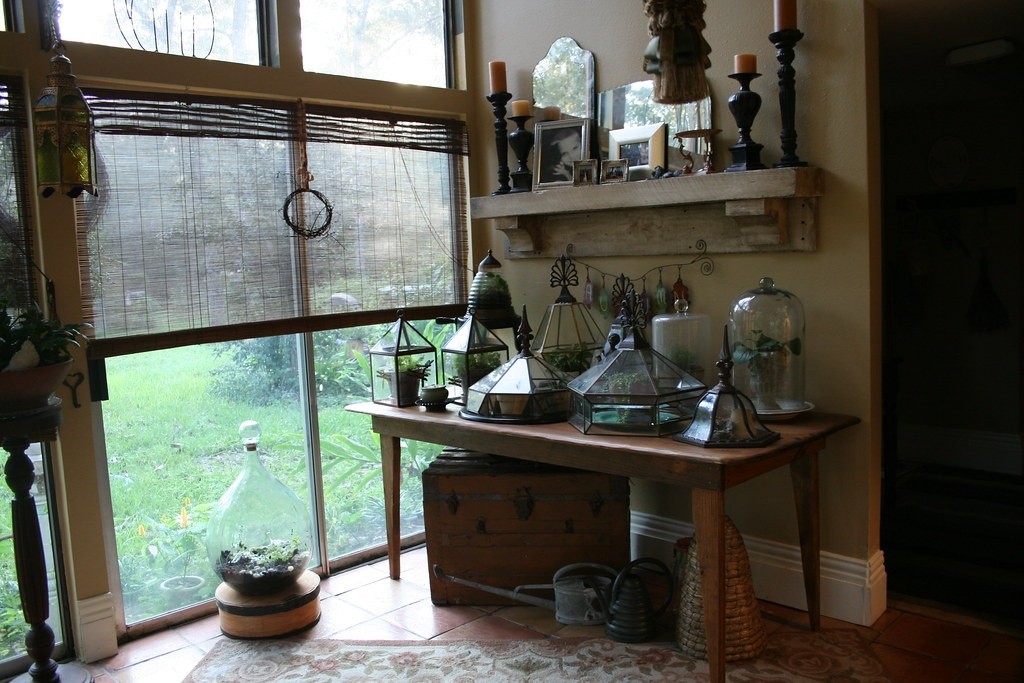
left=30, top=57, right=102, bottom=205
left=543, top=338, right=595, bottom=387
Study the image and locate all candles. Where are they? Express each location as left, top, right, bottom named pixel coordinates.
left=486, top=57, right=509, bottom=96
left=735, top=53, right=757, bottom=73
left=771, top=0, right=799, bottom=30
left=512, top=99, right=530, bottom=116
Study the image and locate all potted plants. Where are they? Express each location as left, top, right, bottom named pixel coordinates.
left=453, top=348, right=505, bottom=400
left=379, top=359, right=434, bottom=404
left=659, top=342, right=708, bottom=397
left=732, top=325, right=813, bottom=409
left=600, top=372, right=668, bottom=423
left=703, top=403, right=767, bottom=440
left=196, top=410, right=318, bottom=595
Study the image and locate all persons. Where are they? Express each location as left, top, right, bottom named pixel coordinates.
left=552, top=128, right=581, bottom=182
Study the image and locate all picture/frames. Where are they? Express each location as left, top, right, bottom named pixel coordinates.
left=608, top=119, right=667, bottom=174
left=533, top=118, right=592, bottom=191
left=571, top=158, right=600, bottom=185
left=600, top=156, right=629, bottom=183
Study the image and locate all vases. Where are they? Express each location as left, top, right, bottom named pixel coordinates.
left=420, top=382, right=450, bottom=403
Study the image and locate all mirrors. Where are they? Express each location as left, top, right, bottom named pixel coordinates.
left=532, top=36, right=596, bottom=119
left=594, top=79, right=717, bottom=172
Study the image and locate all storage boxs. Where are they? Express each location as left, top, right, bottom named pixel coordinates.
left=420, top=445, right=638, bottom=612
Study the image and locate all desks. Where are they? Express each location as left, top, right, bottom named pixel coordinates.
left=341, top=400, right=862, bottom=683
